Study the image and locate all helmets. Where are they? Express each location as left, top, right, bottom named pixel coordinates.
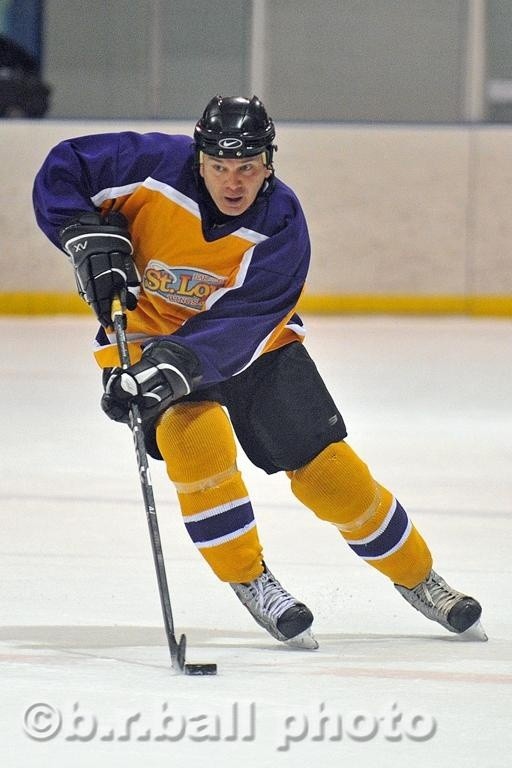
left=193, top=92, right=278, bottom=159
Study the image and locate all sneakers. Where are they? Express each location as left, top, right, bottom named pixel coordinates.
left=394, top=568, right=482, bottom=634
left=228, top=558, right=315, bottom=642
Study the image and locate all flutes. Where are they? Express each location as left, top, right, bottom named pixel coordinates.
left=111, top=292, right=186, bottom=670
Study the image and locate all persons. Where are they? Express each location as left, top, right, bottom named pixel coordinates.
left=28, top=93, right=483, bottom=643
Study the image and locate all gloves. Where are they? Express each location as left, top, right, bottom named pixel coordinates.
left=57, top=210, right=142, bottom=332
left=99, top=337, right=203, bottom=423
